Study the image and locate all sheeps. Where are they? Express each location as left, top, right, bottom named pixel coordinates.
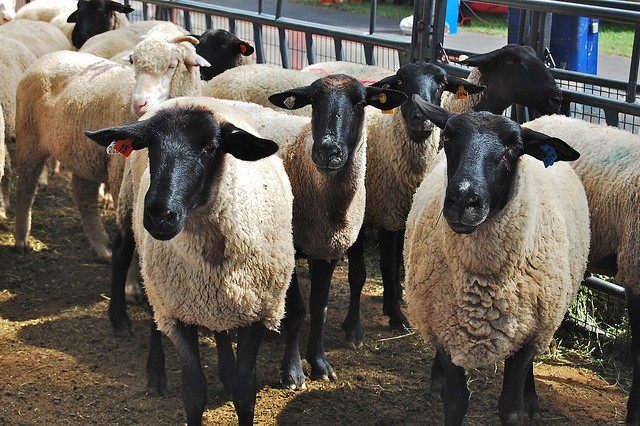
left=105, top=73, right=412, bottom=392
left=1, top=18, right=74, bottom=221
left=14, top=0, right=135, bottom=50
left=77, top=20, right=255, bottom=81
left=301, top=44, right=564, bottom=116
left=401, top=94, right=591, bottom=422
left=524, top=114, right=638, bottom=424
left=203, top=59, right=487, bottom=351
left=14, top=21, right=210, bottom=259
left=83, top=100, right=296, bottom=425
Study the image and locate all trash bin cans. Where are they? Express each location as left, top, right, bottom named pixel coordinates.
left=508, top=7, right=600, bottom=75
